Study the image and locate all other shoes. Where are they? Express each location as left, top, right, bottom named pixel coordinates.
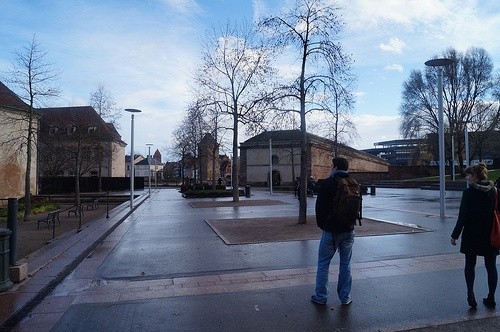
left=342, top=296, right=352, bottom=304
left=311, top=295, right=325, bottom=304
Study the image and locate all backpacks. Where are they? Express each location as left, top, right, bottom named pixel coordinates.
left=328, top=175, right=362, bottom=226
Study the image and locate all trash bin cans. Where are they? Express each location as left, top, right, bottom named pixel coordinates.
left=0, top=227, right=14, bottom=293
left=245, top=183, right=251, bottom=197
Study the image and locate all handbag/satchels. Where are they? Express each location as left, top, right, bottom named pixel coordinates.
left=491, top=210, right=500, bottom=249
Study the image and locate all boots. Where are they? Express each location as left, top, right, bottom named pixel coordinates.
left=467, top=292, right=477, bottom=306
left=483, top=294, right=496, bottom=307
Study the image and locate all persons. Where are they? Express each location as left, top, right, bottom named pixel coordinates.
left=309, top=176, right=315, bottom=197
left=451, top=164, right=500, bottom=308
left=294, top=176, right=299, bottom=197
left=311, top=157, right=355, bottom=305
left=218, top=178, right=222, bottom=185
